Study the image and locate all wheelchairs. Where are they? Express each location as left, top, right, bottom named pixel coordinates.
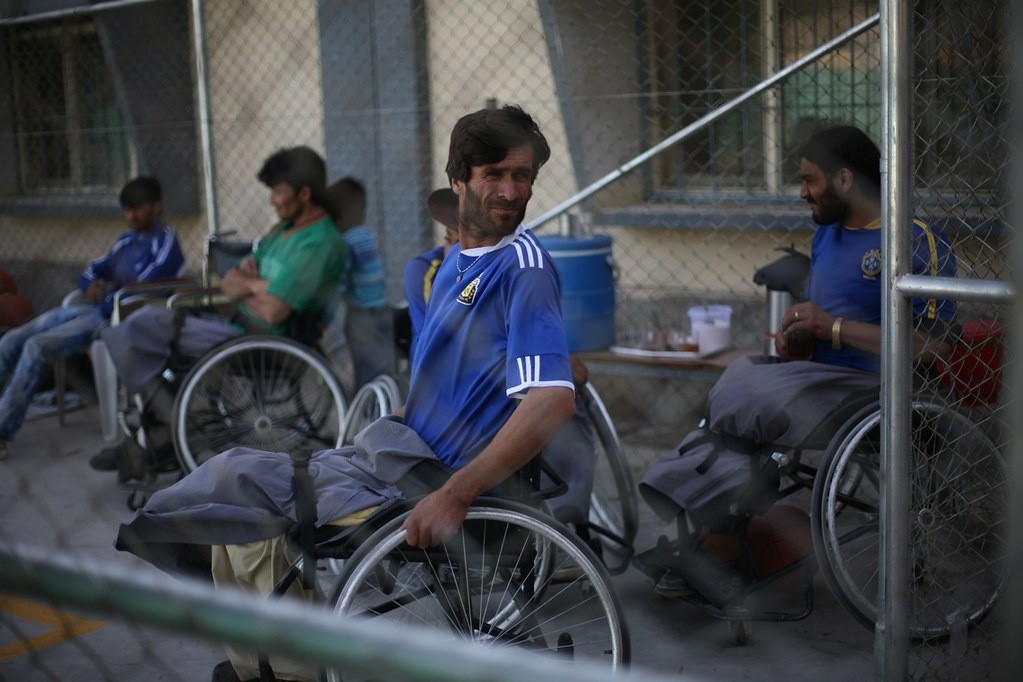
left=118, top=300, right=347, bottom=500
left=314, top=364, right=638, bottom=682
left=638, top=259, right=1012, bottom=644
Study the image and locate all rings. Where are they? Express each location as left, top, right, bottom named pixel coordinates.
left=795, top=312, right=800, bottom=319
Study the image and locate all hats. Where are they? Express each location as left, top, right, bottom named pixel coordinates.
left=427, top=188, right=460, bottom=231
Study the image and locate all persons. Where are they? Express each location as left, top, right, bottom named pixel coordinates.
left=655, top=124, right=954, bottom=598
left=403, top=189, right=595, bottom=578
left=211, top=101, right=580, bottom=682
left=0, top=176, right=185, bottom=455
left=90, top=146, right=347, bottom=473
left=325, top=176, right=397, bottom=420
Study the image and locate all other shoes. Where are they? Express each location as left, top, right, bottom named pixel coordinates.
left=0, top=439, right=8, bottom=458
left=90, top=435, right=173, bottom=471
left=654, top=543, right=763, bottom=602
left=210, top=659, right=262, bottom=682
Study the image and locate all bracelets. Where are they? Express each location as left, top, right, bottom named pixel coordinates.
left=832, top=317, right=844, bottom=350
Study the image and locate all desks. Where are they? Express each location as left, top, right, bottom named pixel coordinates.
left=574, top=339, right=766, bottom=368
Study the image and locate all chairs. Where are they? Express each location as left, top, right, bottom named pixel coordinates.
left=62, top=287, right=192, bottom=442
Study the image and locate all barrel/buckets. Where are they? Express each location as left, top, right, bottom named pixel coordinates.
left=532, top=233, right=621, bottom=353
left=688, top=305, right=733, bottom=357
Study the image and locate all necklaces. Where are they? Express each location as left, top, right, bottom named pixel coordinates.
left=456, top=245, right=494, bottom=283
